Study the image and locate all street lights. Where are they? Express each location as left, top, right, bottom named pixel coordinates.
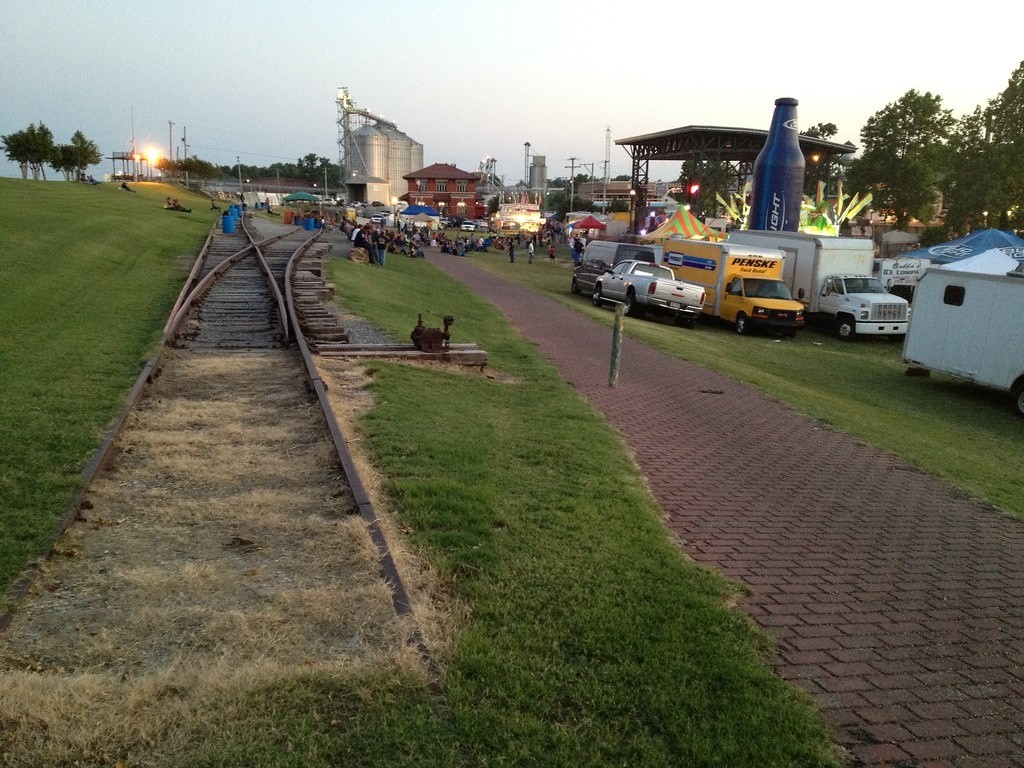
left=391, top=196, right=397, bottom=228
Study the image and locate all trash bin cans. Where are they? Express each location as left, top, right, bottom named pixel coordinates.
left=346, top=208, right=356, bottom=220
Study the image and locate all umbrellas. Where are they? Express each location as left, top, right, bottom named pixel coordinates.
left=408, top=212, right=434, bottom=229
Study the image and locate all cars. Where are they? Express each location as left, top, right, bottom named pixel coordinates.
left=349, top=201, right=385, bottom=208
left=371, top=214, right=382, bottom=224
left=461, top=219, right=488, bottom=232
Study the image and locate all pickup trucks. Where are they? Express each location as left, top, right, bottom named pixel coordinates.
left=592, top=259, right=706, bottom=329
left=322, top=198, right=337, bottom=207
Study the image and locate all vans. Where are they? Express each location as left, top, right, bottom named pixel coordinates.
left=571, top=240, right=656, bottom=295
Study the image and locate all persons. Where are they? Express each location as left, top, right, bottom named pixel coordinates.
left=240, top=192, right=245, bottom=204
left=433, top=232, right=470, bottom=257
left=528, top=239, right=534, bottom=264
left=619, top=232, right=625, bottom=242
left=387, top=219, right=430, bottom=259
left=469, top=233, right=478, bottom=255
left=314, top=211, right=339, bottom=233
left=164, top=197, right=191, bottom=213
left=339, top=216, right=390, bottom=266
left=494, top=224, right=563, bottom=253
left=548, top=244, right=556, bottom=263
left=265, top=198, right=280, bottom=216
left=479, top=235, right=488, bottom=252
left=79, top=173, right=136, bottom=193
left=509, top=242, right=514, bottom=263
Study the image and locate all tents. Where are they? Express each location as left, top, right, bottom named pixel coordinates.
left=283, top=191, right=321, bottom=223
left=896, top=228, right=1024, bottom=269
left=574, top=216, right=608, bottom=241
left=636, top=204, right=730, bottom=245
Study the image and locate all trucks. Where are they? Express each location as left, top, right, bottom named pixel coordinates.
left=661, top=238, right=806, bottom=339
left=729, top=229, right=908, bottom=343
left=872, top=257, right=931, bottom=308
